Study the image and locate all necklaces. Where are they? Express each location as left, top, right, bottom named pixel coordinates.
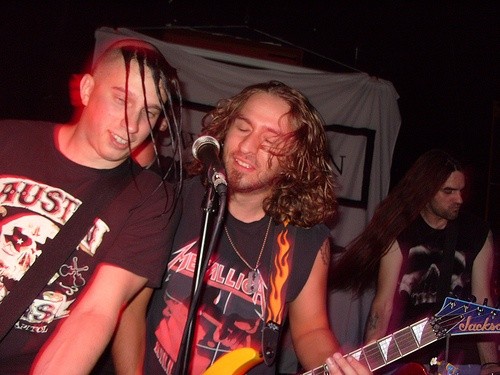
left=224, top=216, right=273, bottom=277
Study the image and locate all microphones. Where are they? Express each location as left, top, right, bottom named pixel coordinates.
left=191, top=135, right=228, bottom=195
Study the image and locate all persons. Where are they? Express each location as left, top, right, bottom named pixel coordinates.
left=334, top=147, right=499, bottom=375
left=1, top=37, right=183, bottom=373
left=112, top=80, right=373, bottom=375
left=134, top=84, right=190, bottom=185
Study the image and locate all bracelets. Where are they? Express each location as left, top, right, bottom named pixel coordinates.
left=481, top=362, right=498, bottom=368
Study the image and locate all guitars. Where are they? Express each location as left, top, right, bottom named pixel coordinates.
left=200, top=291, right=500, bottom=375
